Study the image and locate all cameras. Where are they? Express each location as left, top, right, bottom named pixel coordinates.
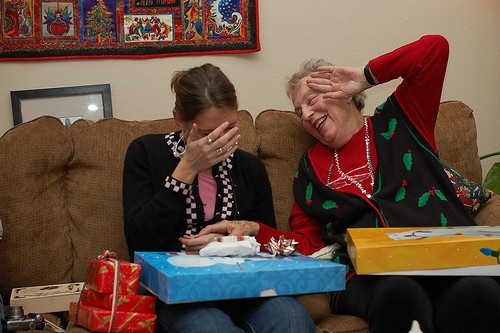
left=2, top=305, right=45, bottom=333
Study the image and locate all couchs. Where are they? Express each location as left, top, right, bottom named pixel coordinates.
left=0, top=99, right=500, bottom=333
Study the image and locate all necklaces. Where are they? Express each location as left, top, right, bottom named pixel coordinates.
left=334, top=118, right=374, bottom=198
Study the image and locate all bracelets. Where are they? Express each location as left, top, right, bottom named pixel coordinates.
left=364, top=64, right=375, bottom=85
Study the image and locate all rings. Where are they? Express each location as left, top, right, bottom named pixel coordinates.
left=216, top=148, right=222, bottom=155
left=207, top=136, right=212, bottom=143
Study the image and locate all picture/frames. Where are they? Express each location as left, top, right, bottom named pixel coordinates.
left=9, top=83, right=113, bottom=126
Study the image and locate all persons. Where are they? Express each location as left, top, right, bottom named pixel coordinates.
left=196, top=34, right=500, bottom=333
left=123, top=64, right=317, bottom=333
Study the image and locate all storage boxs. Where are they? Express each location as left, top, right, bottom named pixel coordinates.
left=11, top=282, right=84, bottom=314
left=347, top=225, right=500, bottom=277
left=134, top=250, right=346, bottom=305
left=81, top=288, right=155, bottom=314
left=68, top=300, right=158, bottom=333
left=87, top=251, right=142, bottom=295
